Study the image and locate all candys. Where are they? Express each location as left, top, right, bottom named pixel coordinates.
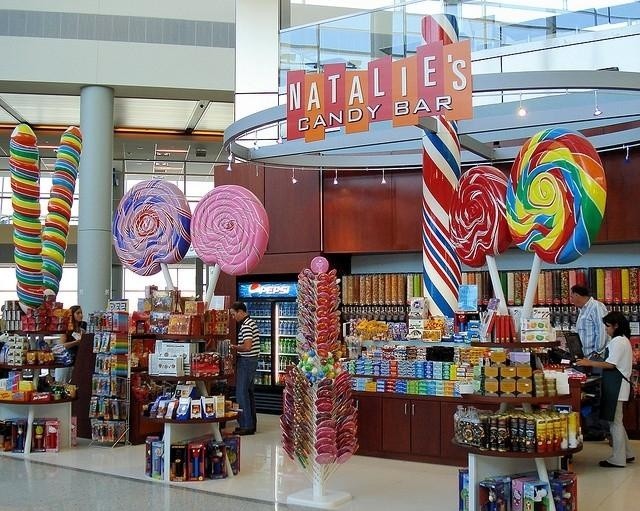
left=112, top=179, right=191, bottom=277
left=506, top=127, right=608, bottom=264
left=43, top=124, right=82, bottom=304
left=9, top=122, right=44, bottom=310
left=280, top=268, right=360, bottom=468
left=448, top=165, right=509, bottom=268
left=190, top=184, right=269, bottom=276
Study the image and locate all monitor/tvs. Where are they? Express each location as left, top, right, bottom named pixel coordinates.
left=561, top=331, right=586, bottom=363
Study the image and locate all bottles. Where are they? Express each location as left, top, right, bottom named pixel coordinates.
left=247, top=302, right=299, bottom=384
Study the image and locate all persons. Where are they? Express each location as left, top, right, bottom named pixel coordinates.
left=229, top=302, right=261, bottom=437
left=569, top=284, right=608, bottom=379
left=56, top=305, right=89, bottom=353
left=574, top=311, right=635, bottom=469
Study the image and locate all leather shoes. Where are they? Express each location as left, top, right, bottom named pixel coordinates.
left=627, top=456, right=635, bottom=463
left=234, top=430, right=254, bottom=434
left=599, top=460, right=625, bottom=468
